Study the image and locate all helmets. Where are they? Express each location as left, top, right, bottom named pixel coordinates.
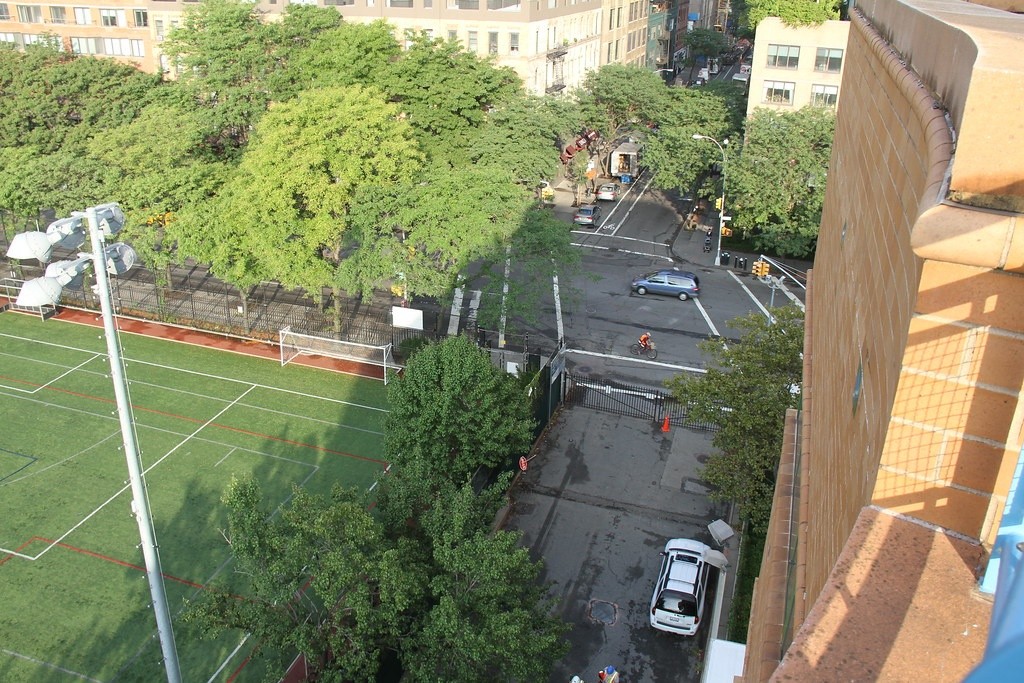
left=572, top=676, right=580, bottom=683
left=607, top=667, right=613, bottom=674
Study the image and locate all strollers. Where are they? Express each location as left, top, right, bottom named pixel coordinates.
left=703, top=236, right=712, bottom=252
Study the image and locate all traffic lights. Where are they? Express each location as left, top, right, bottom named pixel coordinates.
left=726, top=229, right=732, bottom=237
left=716, top=198, right=721, bottom=209
left=755, top=264, right=761, bottom=277
left=751, top=261, right=758, bottom=274
left=763, top=263, right=769, bottom=276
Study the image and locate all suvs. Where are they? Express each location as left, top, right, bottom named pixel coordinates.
left=650, top=538, right=711, bottom=637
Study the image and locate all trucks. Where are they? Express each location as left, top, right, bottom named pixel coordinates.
left=610, top=142, right=646, bottom=179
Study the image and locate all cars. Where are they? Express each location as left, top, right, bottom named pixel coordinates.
left=573, top=204, right=602, bottom=226
left=597, top=183, right=620, bottom=202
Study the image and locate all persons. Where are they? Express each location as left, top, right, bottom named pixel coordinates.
left=600, top=665, right=619, bottom=683
left=637, top=332, right=651, bottom=355
left=706, top=229, right=712, bottom=249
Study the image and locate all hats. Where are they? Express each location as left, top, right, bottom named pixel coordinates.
left=646, top=332, right=651, bottom=336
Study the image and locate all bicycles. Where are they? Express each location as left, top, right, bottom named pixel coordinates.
left=630, top=343, right=657, bottom=360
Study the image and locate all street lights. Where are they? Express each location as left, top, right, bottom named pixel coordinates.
left=6, top=202, right=182, bottom=683
left=693, top=135, right=726, bottom=266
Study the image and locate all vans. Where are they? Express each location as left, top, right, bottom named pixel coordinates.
left=631, top=269, right=700, bottom=301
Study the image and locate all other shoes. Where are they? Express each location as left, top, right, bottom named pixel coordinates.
left=638, top=351, right=640, bottom=355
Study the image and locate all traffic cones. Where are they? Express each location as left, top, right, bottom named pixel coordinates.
left=662, top=416, right=669, bottom=432
